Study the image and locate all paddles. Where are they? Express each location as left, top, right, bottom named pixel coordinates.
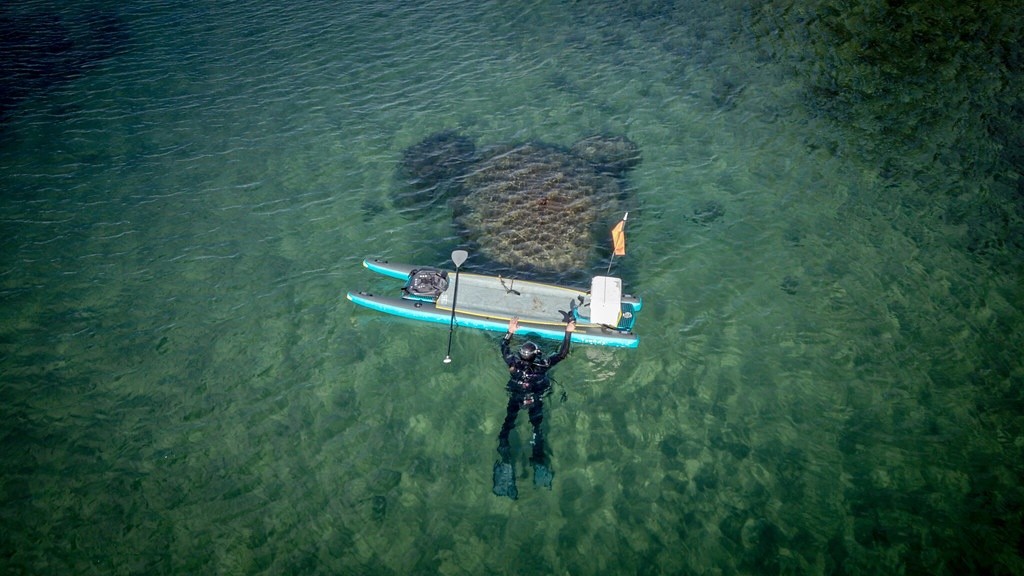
left=442, top=248, right=469, bottom=365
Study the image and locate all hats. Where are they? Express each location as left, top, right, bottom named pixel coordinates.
left=519, top=342, right=538, bottom=361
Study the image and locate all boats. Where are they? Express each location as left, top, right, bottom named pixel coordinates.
left=346, top=211, right=643, bottom=356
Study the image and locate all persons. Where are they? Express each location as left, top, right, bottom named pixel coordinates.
left=491, top=316, right=577, bottom=502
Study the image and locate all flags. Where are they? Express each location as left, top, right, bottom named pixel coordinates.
left=611, top=220, right=626, bottom=255
